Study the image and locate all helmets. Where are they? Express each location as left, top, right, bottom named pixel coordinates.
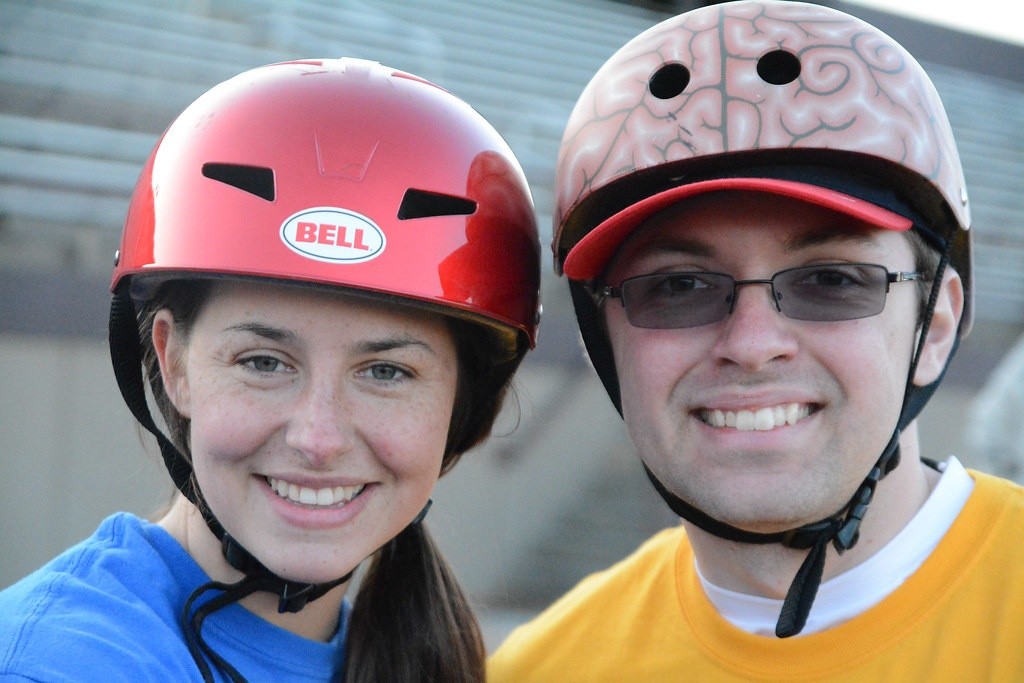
left=551, top=0, right=973, bottom=279
left=109, top=56, right=543, bottom=349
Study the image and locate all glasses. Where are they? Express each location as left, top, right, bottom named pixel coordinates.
left=602, top=262, right=930, bottom=330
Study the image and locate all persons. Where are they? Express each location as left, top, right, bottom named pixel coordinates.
left=0, top=56, right=545, bottom=683
left=486, top=0, right=1023, bottom=683
